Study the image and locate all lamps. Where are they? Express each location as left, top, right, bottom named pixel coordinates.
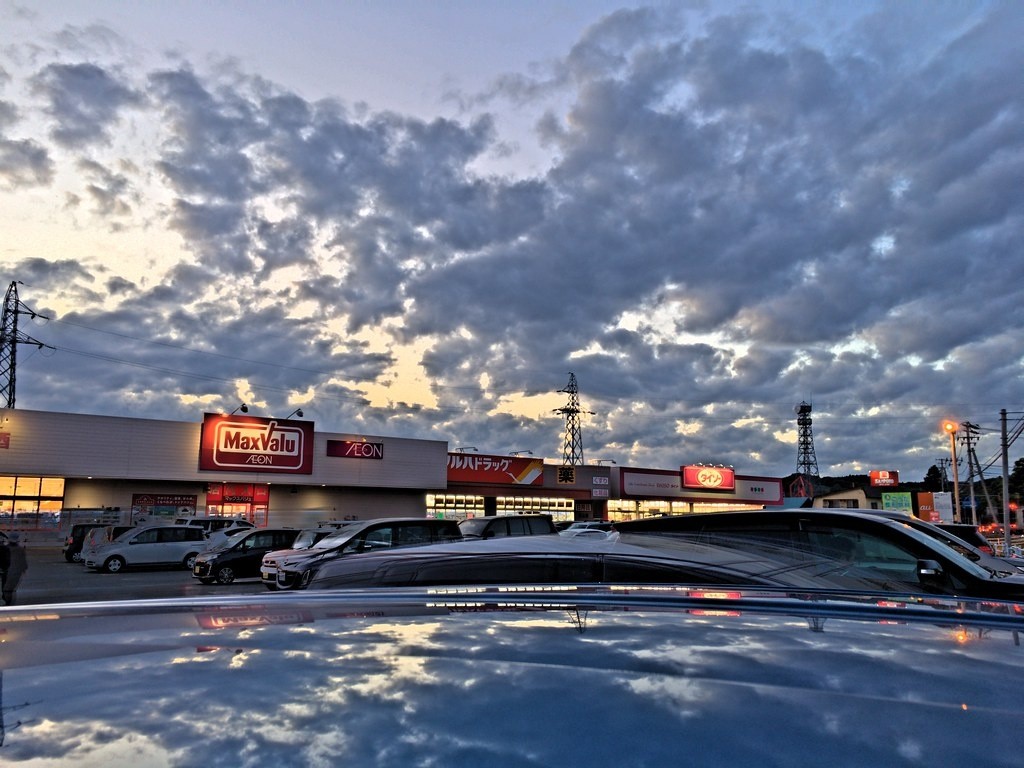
left=287, top=408, right=303, bottom=419
left=231, top=403, right=248, bottom=415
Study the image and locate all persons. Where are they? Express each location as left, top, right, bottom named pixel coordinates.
left=325, top=513, right=360, bottom=530
left=0, top=530, right=27, bottom=607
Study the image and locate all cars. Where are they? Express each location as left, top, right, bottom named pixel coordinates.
left=85, top=521, right=211, bottom=574
left=62, top=508, right=1024, bottom=609
